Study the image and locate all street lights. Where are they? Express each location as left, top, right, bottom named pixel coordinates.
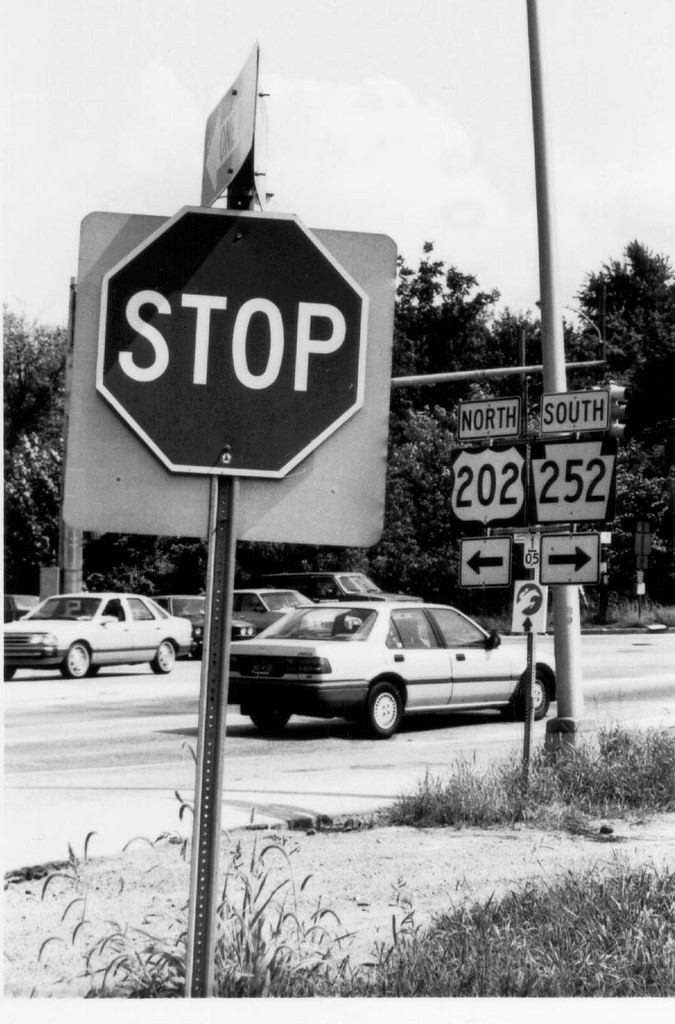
left=536, top=300, right=606, bottom=381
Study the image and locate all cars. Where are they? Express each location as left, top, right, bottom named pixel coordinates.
left=4, top=592, right=193, bottom=681
left=228, top=600, right=557, bottom=740
left=243, top=572, right=425, bottom=634
left=182, top=589, right=362, bottom=636
left=4, top=595, right=40, bottom=623
left=150, top=596, right=256, bottom=661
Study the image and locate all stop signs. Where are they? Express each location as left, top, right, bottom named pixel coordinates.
left=98, top=204, right=368, bottom=480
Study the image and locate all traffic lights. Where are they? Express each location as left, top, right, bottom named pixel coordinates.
left=602, top=381, right=631, bottom=440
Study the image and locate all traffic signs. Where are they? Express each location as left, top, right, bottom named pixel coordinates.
left=201, top=39, right=258, bottom=207
